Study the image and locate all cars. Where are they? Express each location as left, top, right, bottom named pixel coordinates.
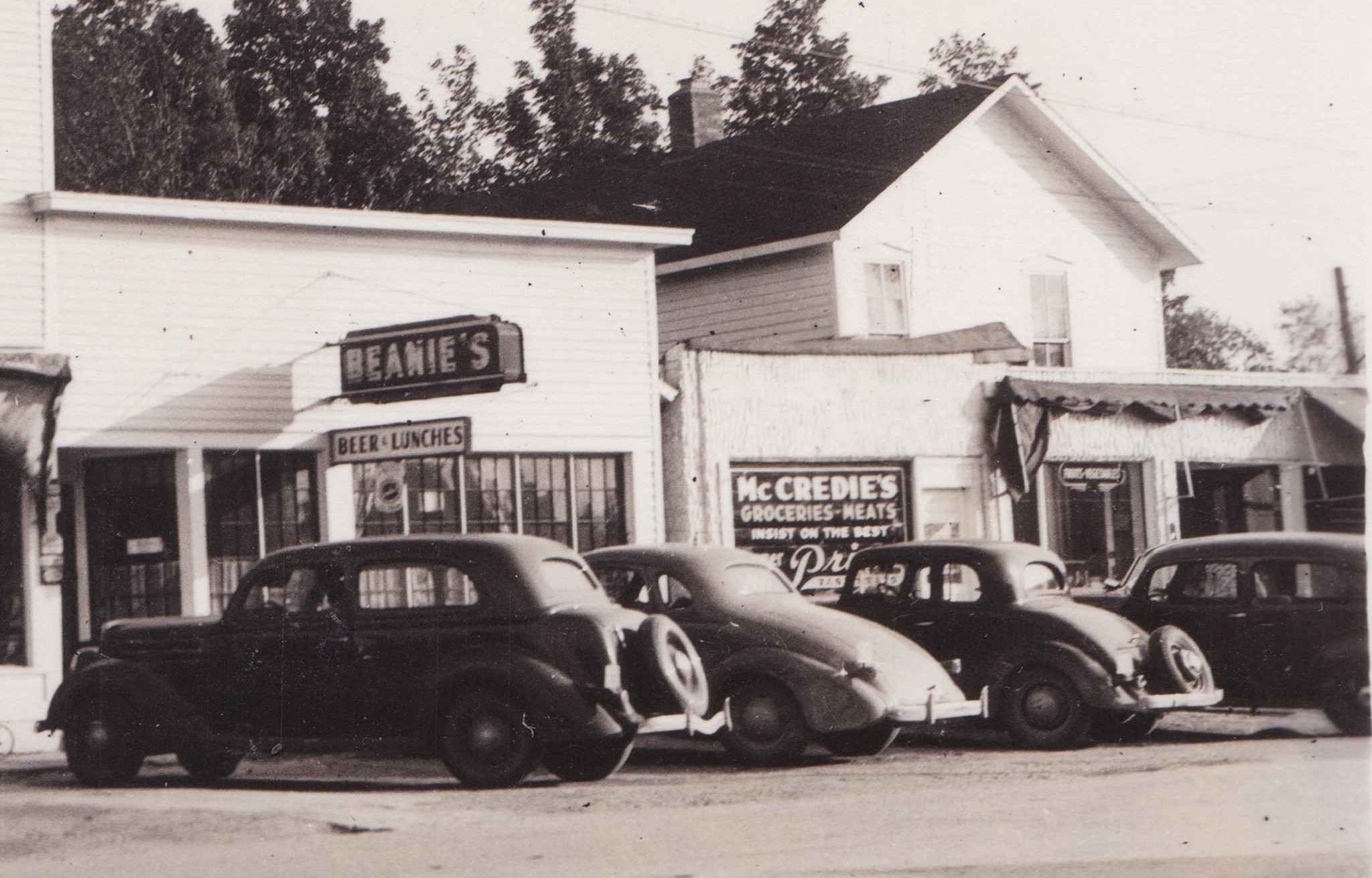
left=37, top=528, right=736, bottom=789
left=1074, top=531, right=1371, bottom=738
left=575, top=539, right=992, bottom=767
left=814, top=536, right=1222, bottom=748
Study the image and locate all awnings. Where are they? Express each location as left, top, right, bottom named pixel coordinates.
left=0, top=347, right=72, bottom=537
left=988, top=376, right=1300, bottom=499
left=1300, top=387, right=1368, bottom=500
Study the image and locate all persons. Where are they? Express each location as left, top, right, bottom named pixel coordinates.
left=324, top=580, right=344, bottom=614
